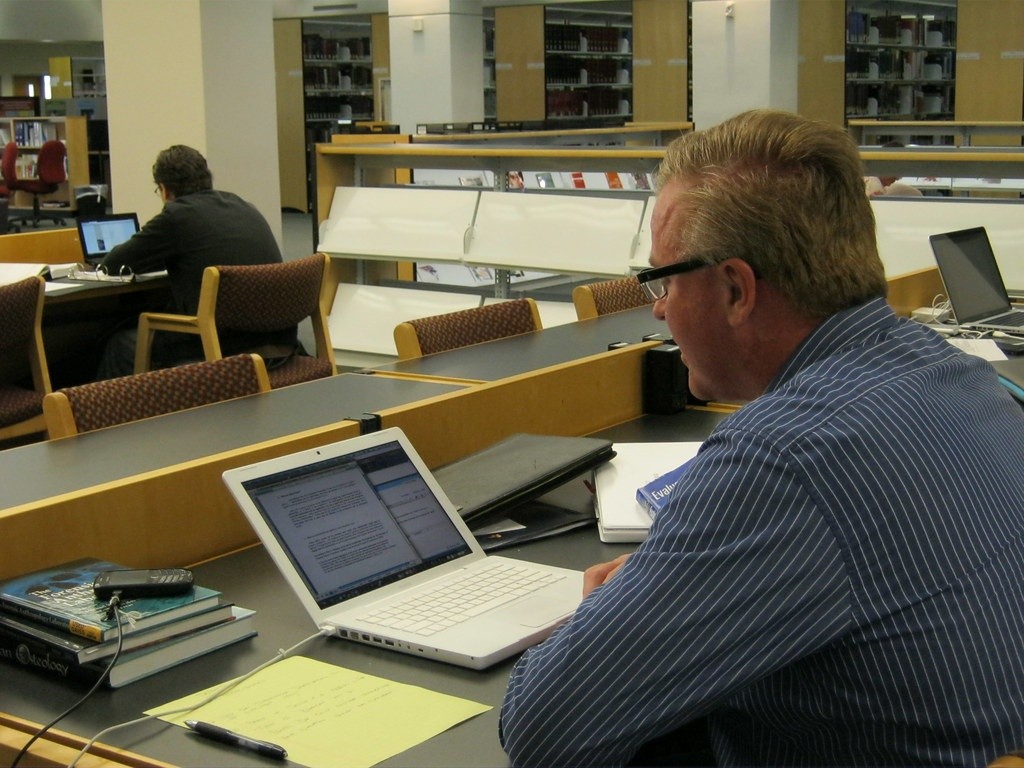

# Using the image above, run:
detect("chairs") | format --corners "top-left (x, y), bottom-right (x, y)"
top-left (0, 254), bottom-right (662, 455)
top-left (12, 138), bottom-right (67, 229)
top-left (0, 141), bottom-right (27, 232)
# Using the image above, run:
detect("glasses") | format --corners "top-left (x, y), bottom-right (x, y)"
top-left (637, 250), bottom-right (763, 302)
top-left (153, 182), bottom-right (172, 198)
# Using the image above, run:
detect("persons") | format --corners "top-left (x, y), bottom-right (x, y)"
top-left (97, 145), bottom-right (299, 380)
top-left (496, 119), bottom-right (1024, 768)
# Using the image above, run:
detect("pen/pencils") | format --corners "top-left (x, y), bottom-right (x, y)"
top-left (183, 720), bottom-right (288, 760)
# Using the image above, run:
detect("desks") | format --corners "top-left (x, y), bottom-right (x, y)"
top-left (43, 267), bottom-right (170, 304)
top-left (1, 374), bottom-right (471, 508)
top-left (1, 409), bottom-right (735, 768)
top-left (362, 300), bottom-right (673, 384)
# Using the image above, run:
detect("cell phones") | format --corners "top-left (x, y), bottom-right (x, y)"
top-left (93, 567), bottom-right (194, 599)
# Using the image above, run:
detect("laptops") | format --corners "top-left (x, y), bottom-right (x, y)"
top-left (221, 427), bottom-right (585, 670)
top-left (76, 212), bottom-right (165, 271)
top-left (928, 227), bottom-right (1024, 335)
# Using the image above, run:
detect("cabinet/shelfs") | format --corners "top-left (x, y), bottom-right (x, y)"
top-left (65, 116), bottom-right (110, 211)
top-left (481, 14), bottom-right (497, 122)
top-left (631, 1), bottom-right (693, 126)
top-left (798, 0), bottom-right (959, 147)
top-left (0, 116), bottom-right (91, 207)
top-left (49, 56), bottom-right (106, 114)
top-left (493, 4), bottom-right (632, 122)
top-left (279, 18), bottom-right (373, 212)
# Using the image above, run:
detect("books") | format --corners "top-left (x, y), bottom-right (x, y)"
top-left (0, 556), bottom-right (259, 687)
top-left (302, 33), bottom-right (374, 200)
top-left (0, 263), bottom-right (78, 286)
top-left (15, 153), bottom-right (68, 180)
top-left (545, 25), bottom-right (619, 118)
top-left (845, 12), bottom-right (957, 145)
top-left (68, 263), bottom-right (168, 282)
top-left (636, 455), bottom-right (695, 522)
top-left (593, 442), bottom-right (705, 544)
top-left (14, 121), bottom-right (57, 147)
